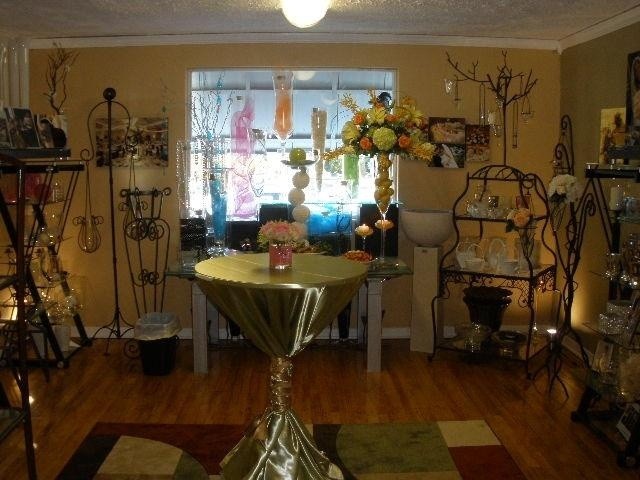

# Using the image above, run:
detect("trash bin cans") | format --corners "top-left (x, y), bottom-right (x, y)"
top-left (134, 312), bottom-right (181, 376)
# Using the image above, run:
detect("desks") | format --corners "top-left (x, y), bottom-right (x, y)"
top-left (166, 251), bottom-right (415, 375)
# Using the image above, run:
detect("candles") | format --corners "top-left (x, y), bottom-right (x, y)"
top-left (359, 223), bottom-right (369, 233)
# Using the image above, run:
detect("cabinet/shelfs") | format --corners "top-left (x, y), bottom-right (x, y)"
top-left (568, 116), bottom-right (637, 468)
top-left (0, 147), bottom-right (93, 480)
top-left (429, 158), bottom-right (554, 381)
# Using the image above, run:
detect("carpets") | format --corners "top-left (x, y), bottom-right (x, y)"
top-left (57, 421), bottom-right (527, 480)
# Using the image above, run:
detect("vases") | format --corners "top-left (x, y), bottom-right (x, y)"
top-left (374, 151), bottom-right (395, 270)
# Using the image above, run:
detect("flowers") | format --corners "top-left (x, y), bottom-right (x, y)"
top-left (319, 86), bottom-right (438, 163)
top-left (256, 219), bottom-right (310, 252)
